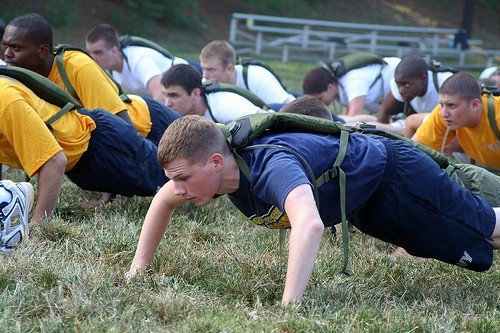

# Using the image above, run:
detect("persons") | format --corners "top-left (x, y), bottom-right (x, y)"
top-left (124, 115), bottom-right (500, 311)
top-left (0, 179), bottom-right (34, 254)
top-left (0, 13), bottom-right (500, 222)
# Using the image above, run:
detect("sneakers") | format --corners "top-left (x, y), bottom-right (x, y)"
top-left (0, 179), bottom-right (34, 255)
